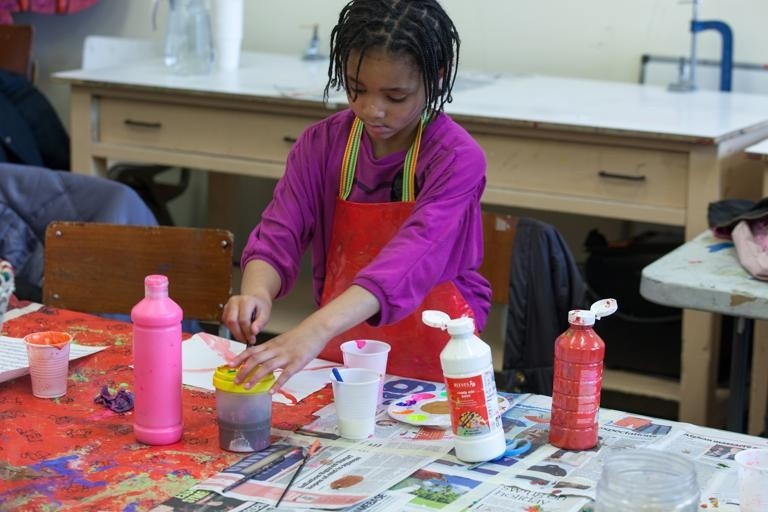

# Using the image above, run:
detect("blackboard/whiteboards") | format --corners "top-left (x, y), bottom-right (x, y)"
top-left (639, 55), bottom-right (768, 94)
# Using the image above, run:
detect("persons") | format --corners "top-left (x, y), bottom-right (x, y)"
top-left (220, 0), bottom-right (492, 395)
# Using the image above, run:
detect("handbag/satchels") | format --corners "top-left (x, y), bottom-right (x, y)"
top-left (580, 229), bottom-right (681, 384)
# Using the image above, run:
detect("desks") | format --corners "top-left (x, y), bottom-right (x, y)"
top-left (0, 294), bottom-right (768, 511)
top-left (635, 224), bottom-right (768, 434)
top-left (45, 61), bottom-right (767, 434)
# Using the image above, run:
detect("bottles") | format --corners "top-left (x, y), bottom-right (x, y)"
top-left (420, 310), bottom-right (507, 464)
top-left (129, 273), bottom-right (184, 445)
top-left (591, 446), bottom-right (702, 512)
top-left (211, 363), bottom-right (273, 454)
top-left (548, 299), bottom-right (621, 455)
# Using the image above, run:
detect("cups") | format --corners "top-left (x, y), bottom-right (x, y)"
top-left (329, 368), bottom-right (379, 441)
top-left (22, 329), bottom-right (69, 399)
top-left (731, 450), bottom-right (768, 512)
top-left (340, 339), bottom-right (391, 408)
top-left (211, 0), bottom-right (246, 85)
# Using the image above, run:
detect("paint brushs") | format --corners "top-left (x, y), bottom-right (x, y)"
top-left (222, 449), bottom-right (301, 493)
top-left (275, 439), bottom-right (321, 508)
top-left (247, 308), bottom-right (256, 349)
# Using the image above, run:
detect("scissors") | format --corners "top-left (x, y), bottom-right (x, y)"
top-left (467, 439), bottom-right (532, 470)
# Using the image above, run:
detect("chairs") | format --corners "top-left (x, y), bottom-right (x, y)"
top-left (476, 207), bottom-right (580, 402)
top-left (41, 218), bottom-right (234, 342)
top-left (0, 21), bottom-right (33, 76)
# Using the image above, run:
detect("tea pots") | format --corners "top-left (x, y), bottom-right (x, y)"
top-left (152, 1), bottom-right (216, 82)
